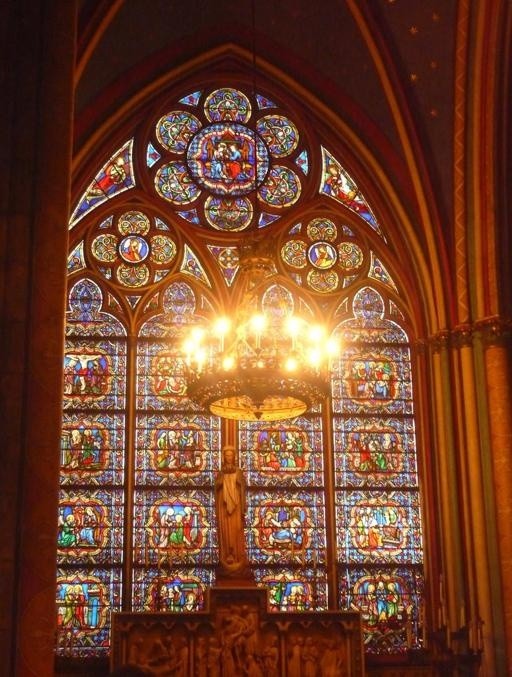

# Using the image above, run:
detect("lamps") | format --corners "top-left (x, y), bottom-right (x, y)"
top-left (182, 3), bottom-right (342, 424)
